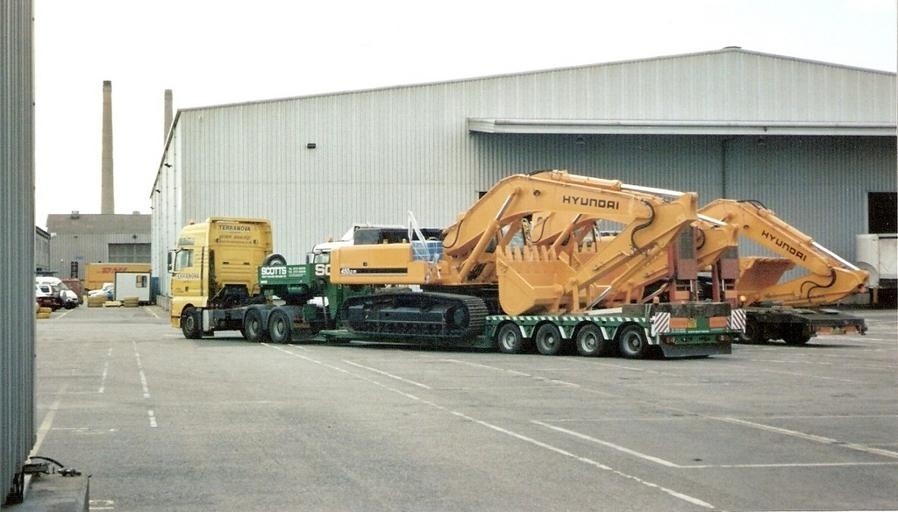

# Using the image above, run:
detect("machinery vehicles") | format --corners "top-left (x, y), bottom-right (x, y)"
top-left (580, 196), bottom-right (871, 315)
top-left (327, 168), bottom-right (742, 319)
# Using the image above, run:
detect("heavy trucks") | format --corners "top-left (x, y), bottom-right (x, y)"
top-left (305, 223), bottom-right (871, 347)
top-left (163, 216), bottom-right (748, 361)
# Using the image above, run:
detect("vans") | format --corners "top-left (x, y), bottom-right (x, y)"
top-left (35, 276), bottom-right (79, 310)
